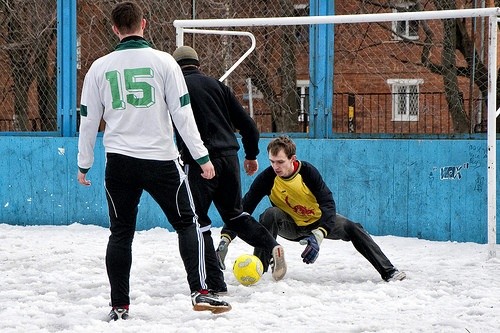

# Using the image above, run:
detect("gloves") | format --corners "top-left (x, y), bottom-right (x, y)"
top-left (215, 238), bottom-right (231, 270)
top-left (299, 228), bottom-right (324, 264)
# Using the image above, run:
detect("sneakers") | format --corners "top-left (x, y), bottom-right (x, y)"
top-left (208, 287), bottom-right (227, 295)
top-left (385, 270), bottom-right (405, 281)
top-left (269, 244), bottom-right (287, 281)
top-left (108, 307), bottom-right (128, 321)
top-left (190, 291), bottom-right (232, 314)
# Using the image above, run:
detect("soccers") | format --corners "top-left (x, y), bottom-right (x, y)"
top-left (233, 255), bottom-right (263, 286)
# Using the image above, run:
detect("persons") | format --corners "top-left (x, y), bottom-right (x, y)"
top-left (172, 46), bottom-right (286, 295)
top-left (216, 137), bottom-right (405, 282)
top-left (77, 2), bottom-right (232, 320)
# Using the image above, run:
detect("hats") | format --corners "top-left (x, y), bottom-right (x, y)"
top-left (173, 46), bottom-right (200, 70)
